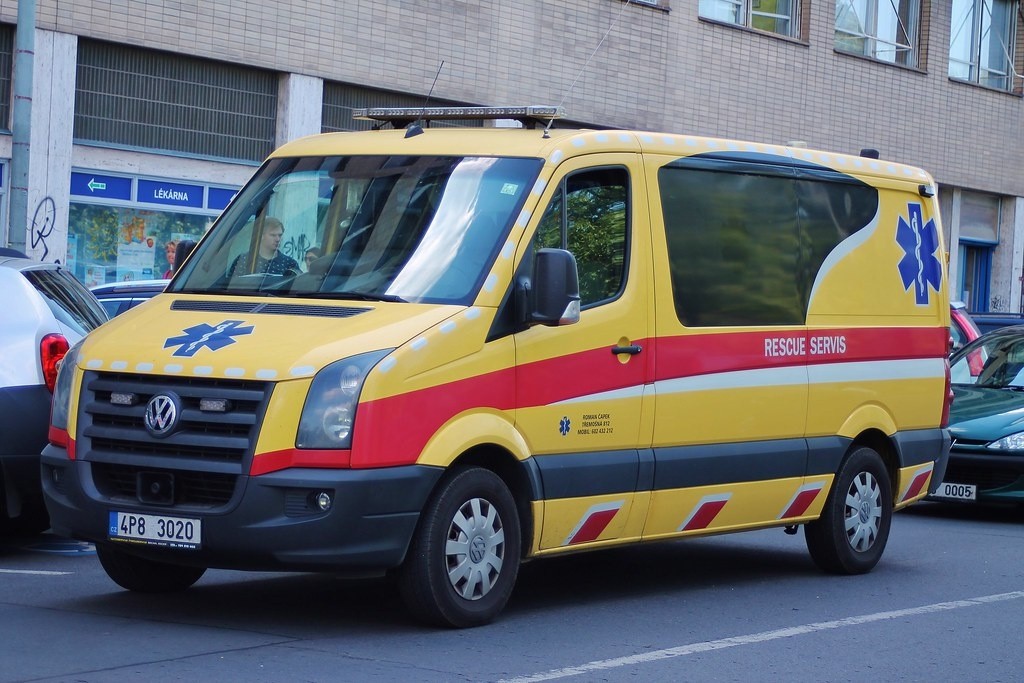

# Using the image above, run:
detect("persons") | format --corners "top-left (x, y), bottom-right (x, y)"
top-left (228, 217), bottom-right (303, 276)
top-left (163, 239), bottom-right (178, 279)
top-left (305, 247), bottom-right (322, 271)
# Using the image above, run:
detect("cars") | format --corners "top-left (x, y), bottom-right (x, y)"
top-left (922, 324), bottom-right (1024, 517)
top-left (946, 301), bottom-right (988, 385)
top-left (89, 278), bottom-right (172, 323)
top-left (0, 250), bottom-right (110, 547)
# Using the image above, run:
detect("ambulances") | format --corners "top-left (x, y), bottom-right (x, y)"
top-left (39, 104), bottom-right (955, 630)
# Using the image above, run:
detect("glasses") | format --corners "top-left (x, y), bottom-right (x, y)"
top-left (305, 256), bottom-right (318, 262)
